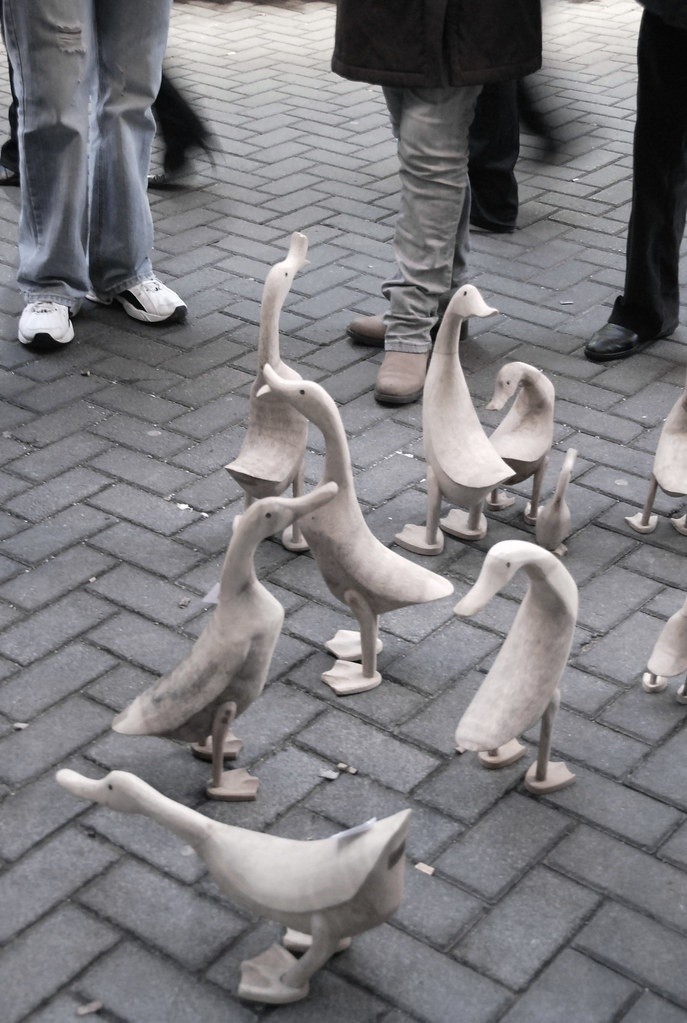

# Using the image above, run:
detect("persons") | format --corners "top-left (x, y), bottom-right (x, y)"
top-left (0, 1), bottom-right (224, 350)
top-left (320, 0), bottom-right (687, 403)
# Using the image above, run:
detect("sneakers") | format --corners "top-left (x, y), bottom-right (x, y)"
top-left (19, 300), bottom-right (75, 350)
top-left (85, 274), bottom-right (188, 326)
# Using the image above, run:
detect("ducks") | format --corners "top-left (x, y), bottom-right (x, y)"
top-left (52, 227), bottom-right (687, 1004)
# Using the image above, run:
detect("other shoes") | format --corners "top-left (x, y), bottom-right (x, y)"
top-left (0, 164), bottom-right (20, 186)
top-left (346, 314), bottom-right (469, 341)
top-left (148, 126), bottom-right (214, 190)
top-left (374, 350), bottom-right (429, 403)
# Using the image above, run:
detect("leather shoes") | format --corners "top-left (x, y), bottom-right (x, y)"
top-left (584, 323), bottom-right (666, 360)
